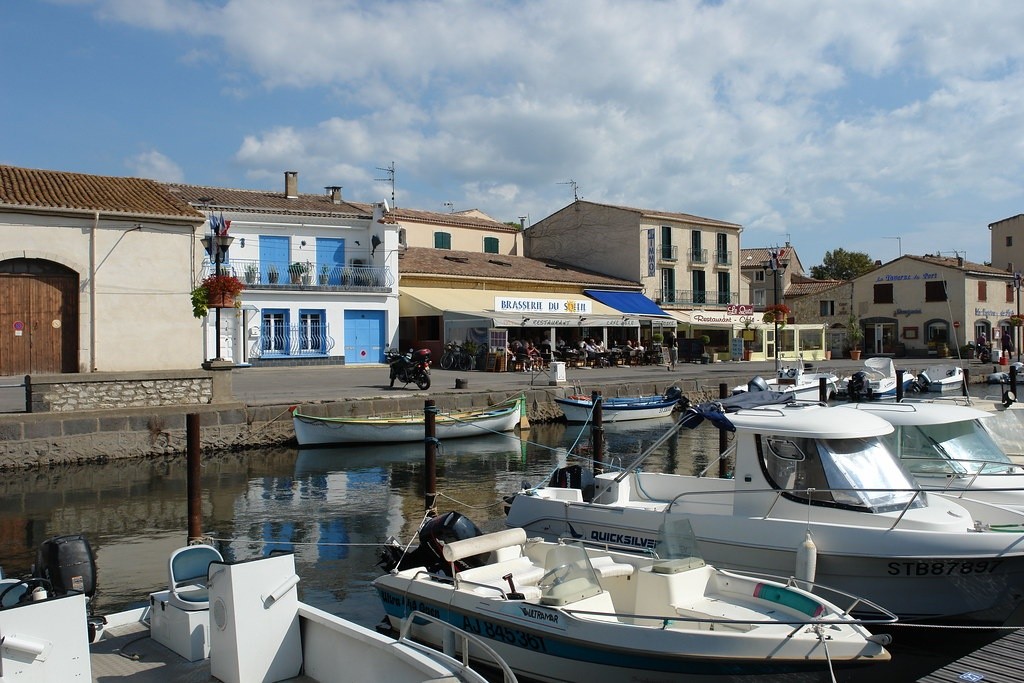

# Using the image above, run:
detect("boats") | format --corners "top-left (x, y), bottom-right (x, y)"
top-left (732, 340), bottom-right (840, 405)
top-left (0, 530), bottom-right (519, 683)
top-left (988, 362), bottom-right (1024, 384)
top-left (838, 356), bottom-right (915, 399)
top-left (555, 386), bottom-right (691, 424)
top-left (291, 394), bottom-right (530, 450)
top-left (505, 388), bottom-right (1024, 652)
top-left (913, 363), bottom-right (965, 392)
top-left (372, 502), bottom-right (899, 683)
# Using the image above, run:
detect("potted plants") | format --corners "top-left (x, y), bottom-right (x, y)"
top-left (319, 264), bottom-right (329, 284)
top-left (244, 265), bottom-right (258, 284)
top-left (844, 314), bottom-right (865, 360)
top-left (266, 264), bottom-right (280, 284)
top-left (221, 264), bottom-right (232, 276)
top-left (354, 272), bottom-right (372, 286)
top-left (959, 336), bottom-right (975, 359)
top-left (459, 339), bottom-right (479, 370)
top-left (826, 339), bottom-right (832, 360)
top-left (286, 262), bottom-right (308, 285)
top-left (744, 346), bottom-right (754, 361)
top-left (301, 261), bottom-right (314, 285)
top-left (651, 333), bottom-right (664, 364)
top-left (341, 267), bottom-right (352, 285)
top-left (698, 334), bottom-right (710, 364)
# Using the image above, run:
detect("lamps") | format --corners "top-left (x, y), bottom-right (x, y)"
top-left (623, 316), bottom-right (630, 322)
top-left (522, 315), bottom-right (530, 322)
top-left (579, 315), bottom-right (586, 322)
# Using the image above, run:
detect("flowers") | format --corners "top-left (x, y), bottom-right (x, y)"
top-left (189, 275), bottom-right (243, 325)
top-left (761, 303), bottom-right (791, 332)
top-left (1010, 314), bottom-right (1024, 327)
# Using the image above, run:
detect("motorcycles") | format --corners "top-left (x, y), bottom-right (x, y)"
top-left (977, 340), bottom-right (990, 362)
top-left (383, 345), bottom-right (432, 390)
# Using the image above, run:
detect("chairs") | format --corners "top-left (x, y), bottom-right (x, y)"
top-left (168, 543), bottom-right (225, 612)
top-left (511, 347), bottom-right (660, 372)
top-left (0, 579), bottom-right (29, 608)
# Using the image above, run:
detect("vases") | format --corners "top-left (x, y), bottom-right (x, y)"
top-left (207, 291), bottom-right (236, 308)
top-left (1011, 319), bottom-right (1024, 326)
top-left (772, 310), bottom-right (783, 322)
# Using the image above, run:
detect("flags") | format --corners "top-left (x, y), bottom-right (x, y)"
top-left (209, 211), bottom-right (225, 232)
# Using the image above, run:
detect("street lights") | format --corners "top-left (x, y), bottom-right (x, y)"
top-left (1005, 273), bottom-right (1024, 362)
top-left (200, 236), bottom-right (235, 360)
top-left (761, 259), bottom-right (790, 372)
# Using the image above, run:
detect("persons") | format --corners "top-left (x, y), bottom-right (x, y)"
top-left (1001, 331), bottom-right (1013, 359)
top-left (506, 335), bottom-right (646, 373)
top-left (977, 332), bottom-right (986, 346)
top-left (668, 332), bottom-right (678, 371)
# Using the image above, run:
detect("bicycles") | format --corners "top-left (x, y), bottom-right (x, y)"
top-left (440, 340), bottom-right (476, 371)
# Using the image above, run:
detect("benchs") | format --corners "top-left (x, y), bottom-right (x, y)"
top-left (675, 591), bottom-right (806, 635)
top-left (443, 527), bottom-right (546, 598)
